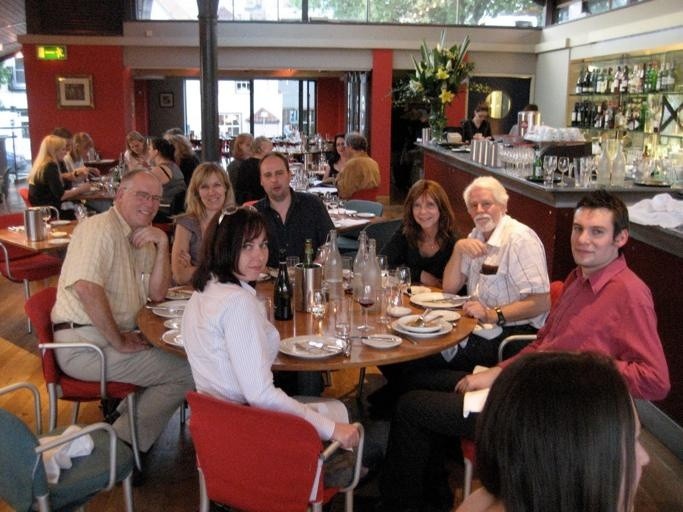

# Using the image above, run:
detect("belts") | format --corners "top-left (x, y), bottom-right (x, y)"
top-left (54, 322), bottom-right (82, 330)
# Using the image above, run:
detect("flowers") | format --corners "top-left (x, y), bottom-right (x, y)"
top-left (380, 25), bottom-right (492, 144)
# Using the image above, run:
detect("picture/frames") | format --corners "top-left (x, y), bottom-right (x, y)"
top-left (158, 91), bottom-right (174, 108)
top-left (55, 72), bottom-right (95, 111)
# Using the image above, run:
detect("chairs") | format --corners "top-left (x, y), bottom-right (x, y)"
top-left (185, 388), bottom-right (365, 512)
top-left (3, 156), bottom-right (414, 272)
top-left (462, 336), bottom-right (539, 506)
top-left (2, 382), bottom-right (134, 512)
top-left (22, 286), bottom-right (190, 481)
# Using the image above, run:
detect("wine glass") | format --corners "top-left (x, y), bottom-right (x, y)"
top-left (39, 206), bottom-right (51, 234)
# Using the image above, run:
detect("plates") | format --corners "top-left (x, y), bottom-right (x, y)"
top-left (50, 219), bottom-right (70, 226)
top-left (151, 284), bottom-right (466, 358)
top-left (50, 231), bottom-right (66, 237)
top-left (46, 239), bottom-right (69, 244)
top-left (356, 212), bottom-right (375, 218)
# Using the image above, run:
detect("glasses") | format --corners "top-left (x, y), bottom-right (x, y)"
top-left (218, 205), bottom-right (257, 225)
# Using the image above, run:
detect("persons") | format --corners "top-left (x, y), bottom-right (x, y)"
top-left (28, 126), bottom-right (670, 510)
top-left (412, 110), bottom-right (430, 140)
top-left (509, 104), bottom-right (538, 135)
top-left (463, 105), bottom-right (494, 144)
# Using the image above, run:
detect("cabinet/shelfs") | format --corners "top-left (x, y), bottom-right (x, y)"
top-left (564, 45), bottom-right (682, 139)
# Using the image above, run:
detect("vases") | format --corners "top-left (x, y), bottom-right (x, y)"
top-left (429, 103), bottom-right (444, 147)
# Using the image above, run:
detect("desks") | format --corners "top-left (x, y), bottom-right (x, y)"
top-left (419, 156), bottom-right (565, 283)
top-left (137, 269), bottom-right (477, 374)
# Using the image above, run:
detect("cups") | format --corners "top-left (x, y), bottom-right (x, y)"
top-left (499, 135), bottom-right (672, 188)
top-left (421, 128), bottom-right (431, 145)
top-left (24, 209), bottom-right (45, 242)
top-left (289, 152), bottom-right (355, 219)
top-left (75, 175), bottom-right (120, 198)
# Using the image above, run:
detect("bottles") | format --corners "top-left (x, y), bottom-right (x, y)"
top-left (570, 95), bottom-right (645, 131)
top-left (273, 228), bottom-right (411, 339)
top-left (575, 59), bottom-right (675, 93)
